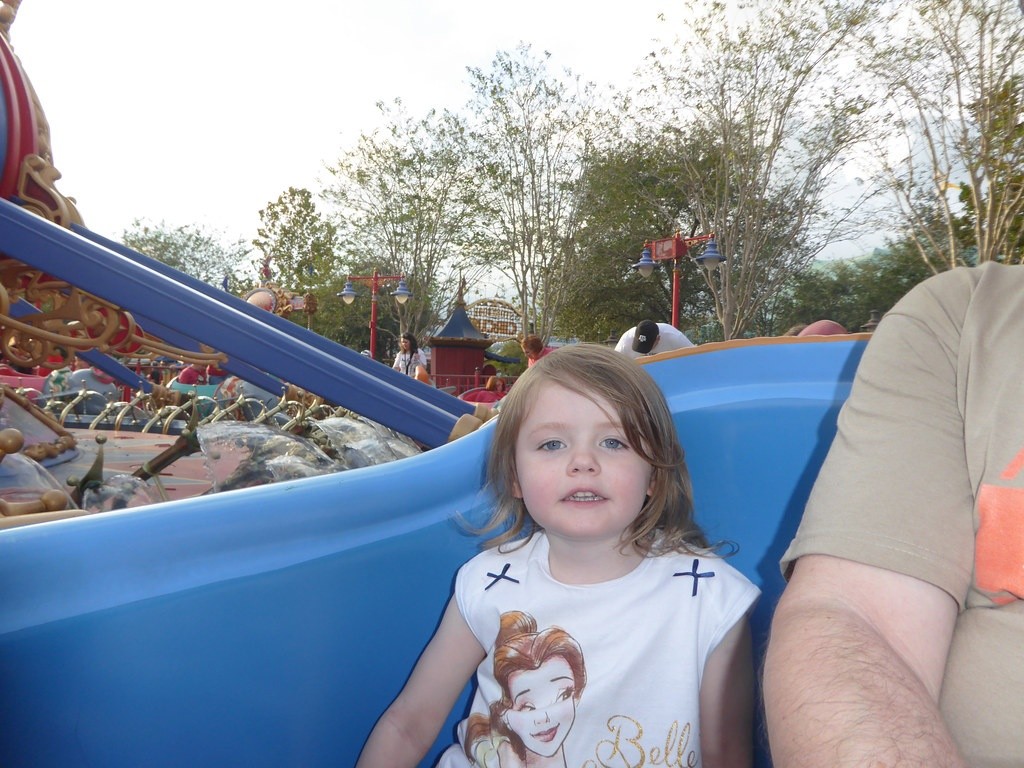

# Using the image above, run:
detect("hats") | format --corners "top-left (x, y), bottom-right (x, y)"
top-left (632, 320), bottom-right (659, 354)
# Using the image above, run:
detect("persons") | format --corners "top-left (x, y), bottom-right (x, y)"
top-left (763, 259), bottom-right (1024, 768)
top-left (360, 332), bottom-right (560, 401)
top-left (614, 319), bottom-right (699, 359)
top-left (356, 343), bottom-right (764, 768)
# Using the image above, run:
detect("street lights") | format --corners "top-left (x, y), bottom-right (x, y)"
top-left (335, 268), bottom-right (420, 365)
top-left (630, 230), bottom-right (728, 331)
